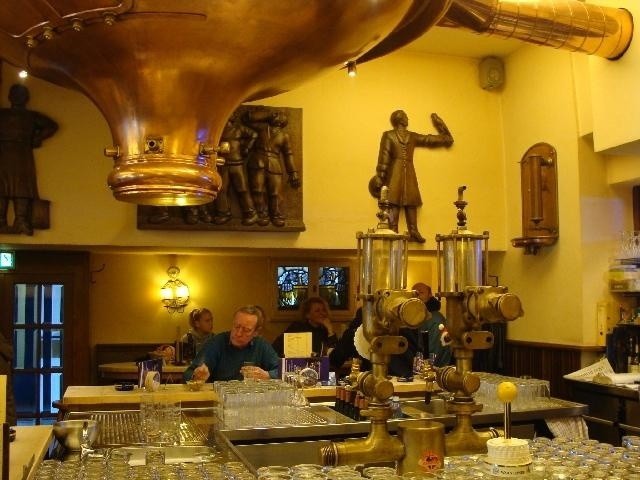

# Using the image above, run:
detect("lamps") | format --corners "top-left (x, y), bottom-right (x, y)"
top-left (0, 252), bottom-right (14, 269)
top-left (347, 58), bottom-right (358, 78)
top-left (158, 263), bottom-right (192, 313)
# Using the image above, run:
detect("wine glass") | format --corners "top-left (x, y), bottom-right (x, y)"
top-left (614, 229), bottom-right (640, 258)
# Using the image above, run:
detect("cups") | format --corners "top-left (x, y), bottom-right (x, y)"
top-left (213, 361), bottom-right (299, 427)
top-left (140, 393), bottom-right (182, 443)
top-left (33, 436), bottom-right (640, 480)
top-left (472, 372), bottom-right (551, 412)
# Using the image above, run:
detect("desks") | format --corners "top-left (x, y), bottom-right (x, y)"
top-left (0, 361), bottom-right (440, 480)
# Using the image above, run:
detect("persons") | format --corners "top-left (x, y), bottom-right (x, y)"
top-left (172, 306), bottom-right (218, 363)
top-left (329, 302), bottom-right (416, 378)
top-left (147, 205), bottom-right (200, 224)
top-left (0, 83), bottom-right (60, 233)
top-left (211, 110), bottom-right (263, 225)
top-left (183, 305), bottom-right (283, 380)
top-left (241, 104), bottom-right (301, 228)
top-left (272, 296), bottom-right (338, 359)
top-left (406, 283), bottom-right (454, 367)
top-left (377, 109), bottom-right (454, 242)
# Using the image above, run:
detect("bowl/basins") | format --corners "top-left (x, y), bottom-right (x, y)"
top-left (185, 379), bottom-right (205, 392)
top-left (53, 419), bottom-right (101, 452)
